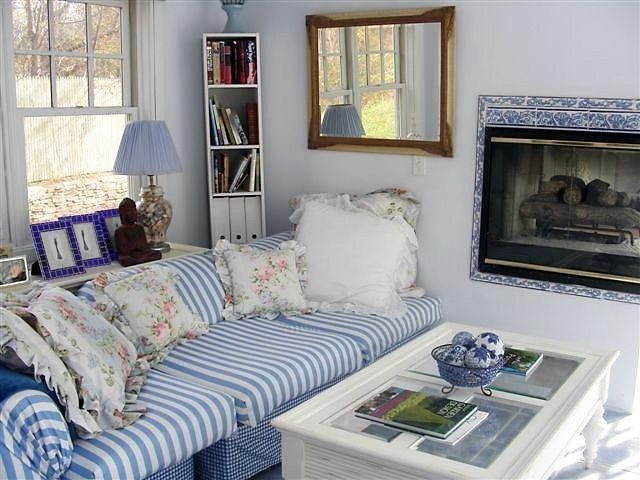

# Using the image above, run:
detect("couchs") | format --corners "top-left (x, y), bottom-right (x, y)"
top-left (0, 228), bottom-right (444, 480)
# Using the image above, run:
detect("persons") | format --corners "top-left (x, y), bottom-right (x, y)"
top-left (115, 197), bottom-right (163, 267)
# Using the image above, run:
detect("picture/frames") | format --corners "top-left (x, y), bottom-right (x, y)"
top-left (95, 209), bottom-right (122, 261)
top-left (29, 220), bottom-right (86, 280)
top-left (58, 213), bottom-right (111, 269)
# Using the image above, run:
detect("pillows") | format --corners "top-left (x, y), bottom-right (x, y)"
top-left (9, 282), bottom-right (150, 429)
top-left (94, 271), bottom-right (209, 367)
top-left (0, 299), bottom-right (102, 441)
top-left (213, 239), bottom-right (315, 321)
top-left (288, 186), bottom-right (421, 318)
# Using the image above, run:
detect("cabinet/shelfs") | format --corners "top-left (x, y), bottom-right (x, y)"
top-left (202, 32), bottom-right (267, 249)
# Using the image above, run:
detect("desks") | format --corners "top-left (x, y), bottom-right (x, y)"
top-left (271, 320), bottom-right (619, 480)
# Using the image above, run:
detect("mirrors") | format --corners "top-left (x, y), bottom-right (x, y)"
top-left (305, 5), bottom-right (455, 159)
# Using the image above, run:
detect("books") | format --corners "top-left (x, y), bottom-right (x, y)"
top-left (353, 385), bottom-right (491, 446)
top-left (499, 346), bottom-right (543, 378)
top-left (206, 39), bottom-right (260, 197)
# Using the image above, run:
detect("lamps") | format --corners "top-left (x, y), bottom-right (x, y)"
top-left (113, 120), bottom-right (180, 252)
top-left (319, 104), bottom-right (366, 138)
